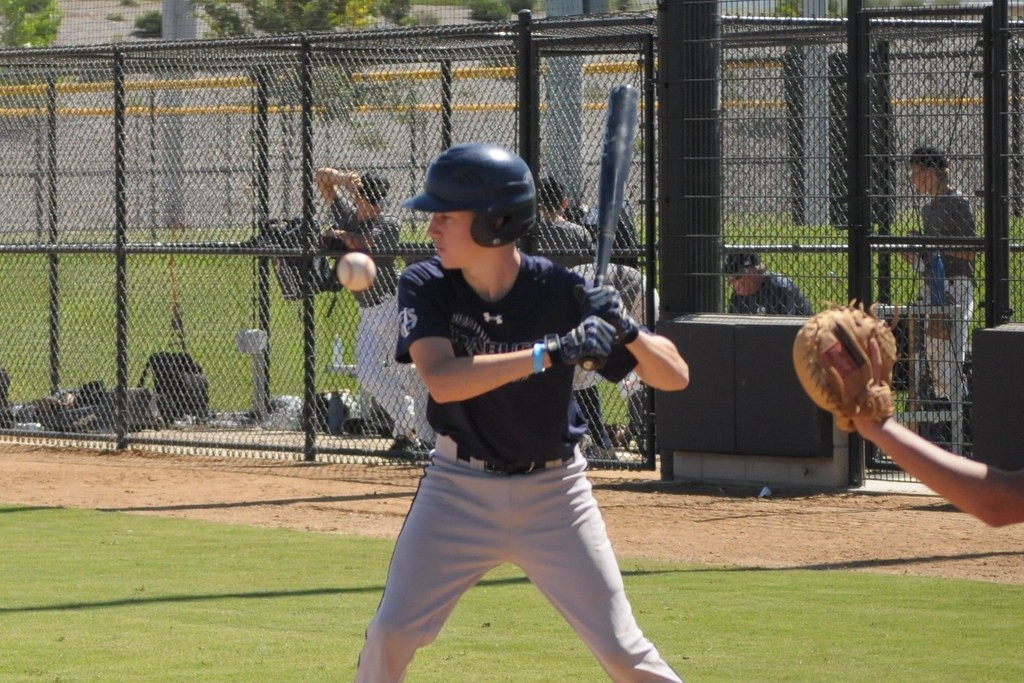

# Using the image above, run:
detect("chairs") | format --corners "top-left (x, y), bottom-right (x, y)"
top-left (873, 304), bottom-right (963, 456)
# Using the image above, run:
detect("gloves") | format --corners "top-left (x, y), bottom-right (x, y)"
top-left (533, 315), bottom-right (616, 374)
top-left (573, 284), bottom-right (638, 344)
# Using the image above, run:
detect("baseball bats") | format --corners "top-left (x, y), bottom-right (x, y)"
top-left (575, 84), bottom-right (640, 370)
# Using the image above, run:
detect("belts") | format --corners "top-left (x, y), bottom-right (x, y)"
top-left (456, 443), bottom-right (574, 477)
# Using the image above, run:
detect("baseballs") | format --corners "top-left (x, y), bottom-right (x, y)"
top-left (336, 252), bottom-right (377, 292)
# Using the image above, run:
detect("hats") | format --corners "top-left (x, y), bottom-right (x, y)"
top-left (359, 172), bottom-right (391, 208)
top-left (719, 247), bottom-right (762, 278)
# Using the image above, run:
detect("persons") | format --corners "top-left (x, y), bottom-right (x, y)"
top-left (314, 168), bottom-right (436, 452)
top-left (536, 175), bottom-right (658, 457)
top-left (352, 143), bottom-right (690, 683)
top-left (832, 336), bottom-right (1024, 527)
top-left (901, 146), bottom-right (975, 400)
top-left (722, 252), bottom-right (811, 316)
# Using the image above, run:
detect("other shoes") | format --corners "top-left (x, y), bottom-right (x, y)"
top-left (385, 437), bottom-right (412, 456)
top-left (413, 440), bottom-right (438, 454)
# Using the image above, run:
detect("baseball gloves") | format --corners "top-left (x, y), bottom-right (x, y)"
top-left (790, 305), bottom-right (898, 431)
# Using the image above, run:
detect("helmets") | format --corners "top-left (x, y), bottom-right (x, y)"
top-left (399, 143), bottom-right (537, 248)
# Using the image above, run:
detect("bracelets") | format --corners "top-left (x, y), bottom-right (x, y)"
top-left (531, 342), bottom-right (544, 375)
